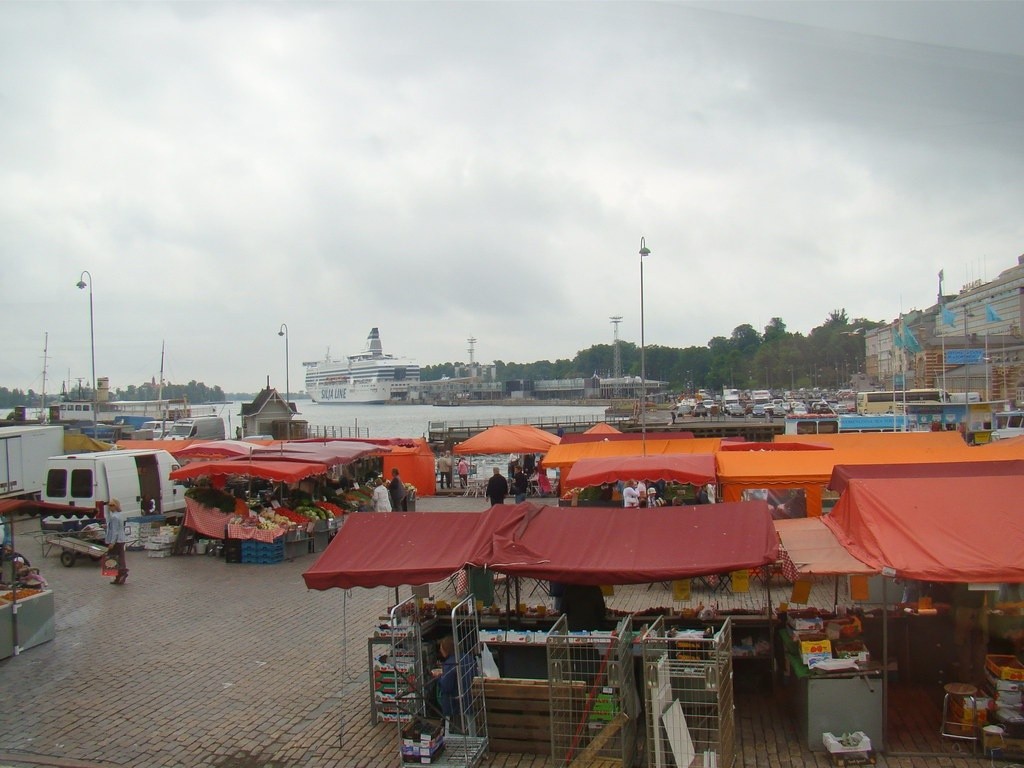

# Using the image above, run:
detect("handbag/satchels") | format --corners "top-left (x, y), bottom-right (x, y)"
top-left (102, 550), bottom-right (119, 576)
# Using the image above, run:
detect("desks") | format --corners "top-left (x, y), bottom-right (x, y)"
top-left (478, 641), bottom-right (595, 646)
top-left (474, 479), bottom-right (490, 497)
top-left (436, 616), bottom-right (567, 672)
top-left (866, 611), bottom-right (956, 683)
top-left (778, 628), bottom-right (883, 751)
top-left (610, 613), bottom-right (781, 704)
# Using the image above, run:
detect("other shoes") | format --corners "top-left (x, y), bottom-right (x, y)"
top-left (110, 573), bottom-right (128, 584)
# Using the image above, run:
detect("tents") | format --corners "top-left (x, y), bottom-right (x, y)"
top-left (165, 423), bottom-right (1024, 758)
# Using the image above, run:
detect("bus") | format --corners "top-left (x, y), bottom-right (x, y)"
top-left (856, 389), bottom-right (952, 416)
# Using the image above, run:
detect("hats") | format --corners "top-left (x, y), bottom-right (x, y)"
top-left (647, 488), bottom-right (656, 495)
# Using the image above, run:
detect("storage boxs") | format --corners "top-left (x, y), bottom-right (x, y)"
top-left (479, 629), bottom-right (725, 676)
top-left (786, 608), bottom-right (871, 665)
top-left (225, 505), bottom-right (369, 564)
top-left (731, 637), bottom-right (773, 661)
top-left (886, 656), bottom-right (899, 682)
top-left (822, 731), bottom-right (877, 765)
top-left (373, 612), bottom-right (437, 724)
top-left (587, 692), bottom-right (620, 720)
top-left (949, 654), bottom-right (1024, 760)
top-left (401, 715), bottom-right (445, 764)
top-left (79, 515), bottom-right (181, 558)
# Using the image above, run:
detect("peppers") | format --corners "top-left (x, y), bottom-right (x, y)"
top-left (274, 507), bottom-right (307, 523)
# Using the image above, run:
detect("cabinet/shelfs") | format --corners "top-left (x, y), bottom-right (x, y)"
top-left (368, 621), bottom-right (439, 725)
top-left (642, 614), bottom-right (738, 768)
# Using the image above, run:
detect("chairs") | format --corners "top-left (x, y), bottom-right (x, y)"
top-left (460, 478), bottom-right (476, 496)
top-left (529, 478), bottom-right (542, 497)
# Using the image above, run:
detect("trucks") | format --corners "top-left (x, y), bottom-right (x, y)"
top-left (0, 424), bottom-right (64, 500)
top-left (723, 389), bottom-right (740, 395)
top-left (751, 390), bottom-right (770, 405)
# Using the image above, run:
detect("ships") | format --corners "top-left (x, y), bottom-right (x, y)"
top-left (305, 327), bottom-right (420, 404)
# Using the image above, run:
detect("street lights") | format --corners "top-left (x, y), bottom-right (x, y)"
top-left (639, 237), bottom-right (650, 457)
top-left (963, 303), bottom-right (976, 442)
top-left (77, 270), bottom-right (97, 441)
top-left (279, 323), bottom-right (290, 441)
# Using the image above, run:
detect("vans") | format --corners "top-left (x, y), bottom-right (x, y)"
top-left (991, 412), bottom-right (1024, 439)
top-left (138, 420), bottom-right (175, 431)
top-left (41, 448), bottom-right (195, 533)
top-left (724, 395), bottom-right (740, 412)
top-left (163, 415), bottom-right (225, 441)
top-left (243, 435), bottom-right (275, 441)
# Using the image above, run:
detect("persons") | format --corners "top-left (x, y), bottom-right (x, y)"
top-left (511, 465), bottom-right (529, 503)
top-left (537, 455), bottom-right (552, 493)
top-left (430, 635), bottom-right (477, 734)
top-left (699, 483), bottom-right (711, 504)
top-left (437, 449), bottom-right (451, 488)
top-left (485, 467), bottom-right (508, 508)
top-left (443, 450), bottom-right (454, 488)
top-left (390, 467), bottom-right (404, 511)
top-left (707, 482), bottom-right (716, 504)
top-left (646, 487), bottom-right (657, 508)
top-left (370, 478), bottom-right (392, 512)
top-left (105, 499), bottom-right (129, 584)
top-left (509, 452), bottom-right (520, 465)
top-left (458, 456), bottom-right (468, 488)
top-left (623, 479), bottom-right (640, 508)
top-left (638, 479), bottom-right (647, 506)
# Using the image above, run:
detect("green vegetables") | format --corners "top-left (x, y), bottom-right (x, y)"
top-left (184, 486), bottom-right (237, 515)
top-left (288, 488), bottom-right (329, 521)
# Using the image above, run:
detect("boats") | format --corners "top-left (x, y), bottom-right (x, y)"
top-left (325, 377), bottom-right (347, 383)
top-left (56, 378), bottom-right (192, 423)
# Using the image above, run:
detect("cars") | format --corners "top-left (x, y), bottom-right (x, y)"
top-left (154, 431), bottom-right (169, 440)
top-left (677, 388), bottom-right (858, 416)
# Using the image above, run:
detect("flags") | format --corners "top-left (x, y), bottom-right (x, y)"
top-left (894, 329), bottom-right (902, 348)
top-left (985, 307), bottom-right (1000, 323)
top-left (941, 307), bottom-right (958, 327)
top-left (903, 323), bottom-right (922, 353)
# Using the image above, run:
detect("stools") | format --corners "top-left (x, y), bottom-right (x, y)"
top-left (444, 698), bottom-right (482, 735)
top-left (981, 724), bottom-right (1007, 767)
top-left (940, 683), bottom-right (978, 754)
